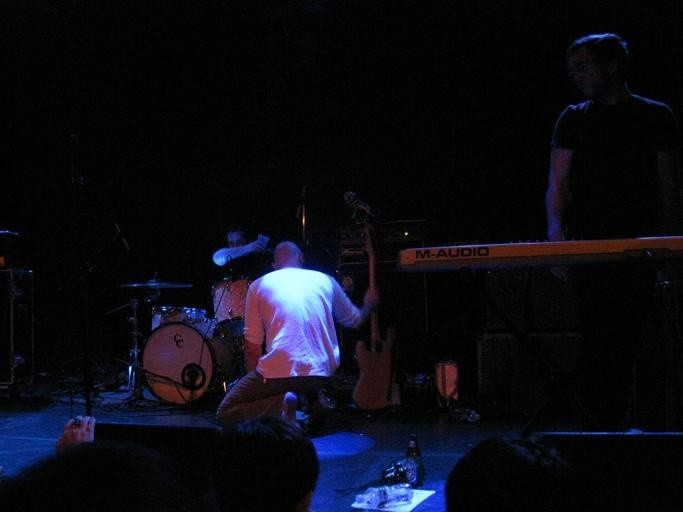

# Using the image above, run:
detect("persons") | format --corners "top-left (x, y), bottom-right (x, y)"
top-left (444, 430), bottom-right (581, 510)
top-left (211, 238), bottom-right (378, 436)
top-left (210, 417), bottom-right (319, 512)
top-left (544, 32), bottom-right (682, 426)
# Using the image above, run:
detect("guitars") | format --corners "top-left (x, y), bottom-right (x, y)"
top-left (352, 222), bottom-right (404, 410)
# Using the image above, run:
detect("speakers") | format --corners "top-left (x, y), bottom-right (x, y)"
top-left (0, 269), bottom-right (35, 385)
top-left (337, 258), bottom-right (432, 390)
top-left (96, 424), bottom-right (219, 467)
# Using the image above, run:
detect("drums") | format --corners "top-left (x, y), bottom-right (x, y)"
top-left (150, 304), bottom-right (179, 330)
top-left (217, 316), bottom-right (244, 354)
top-left (139, 319), bottom-right (232, 408)
top-left (177, 305), bottom-right (206, 333)
top-left (211, 276), bottom-right (259, 325)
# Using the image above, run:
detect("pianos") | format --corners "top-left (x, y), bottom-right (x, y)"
top-left (400, 232), bottom-right (683, 274)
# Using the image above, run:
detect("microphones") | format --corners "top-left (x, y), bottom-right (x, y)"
top-left (344, 191), bottom-right (372, 213)
top-left (109, 214), bottom-right (129, 251)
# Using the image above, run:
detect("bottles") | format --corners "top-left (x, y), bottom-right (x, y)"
top-left (354, 482), bottom-right (414, 509)
top-left (406, 432), bottom-right (425, 487)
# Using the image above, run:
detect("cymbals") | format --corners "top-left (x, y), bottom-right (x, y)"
top-left (117, 279), bottom-right (192, 290)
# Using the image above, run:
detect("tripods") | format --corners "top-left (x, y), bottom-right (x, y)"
top-left (105, 293), bottom-right (163, 411)
